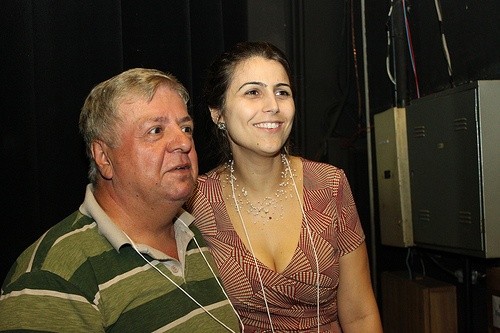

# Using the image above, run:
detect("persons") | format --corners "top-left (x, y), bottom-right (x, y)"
top-left (2, 69), bottom-right (243, 333)
top-left (184, 40), bottom-right (383, 332)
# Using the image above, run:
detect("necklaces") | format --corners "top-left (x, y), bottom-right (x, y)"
top-left (119, 216), bottom-right (243, 332)
top-left (223, 154), bottom-right (321, 333)
